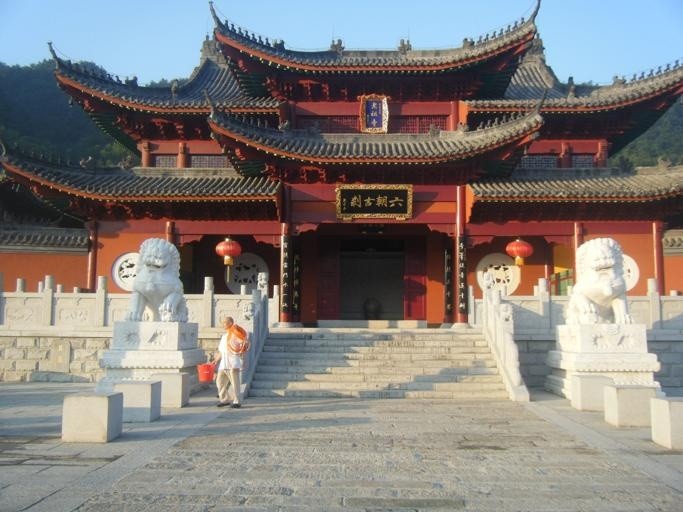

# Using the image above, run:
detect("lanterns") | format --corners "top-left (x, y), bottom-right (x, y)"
top-left (215, 237), bottom-right (242, 283)
top-left (505, 237), bottom-right (534, 266)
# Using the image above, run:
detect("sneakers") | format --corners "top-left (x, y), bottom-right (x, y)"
top-left (216, 403), bottom-right (241, 408)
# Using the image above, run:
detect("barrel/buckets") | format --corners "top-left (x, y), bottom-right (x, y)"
top-left (227, 325), bottom-right (249, 356)
top-left (196, 362), bottom-right (217, 382)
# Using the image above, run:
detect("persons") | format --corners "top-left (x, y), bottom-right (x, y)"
top-left (211, 316), bottom-right (241, 408)
